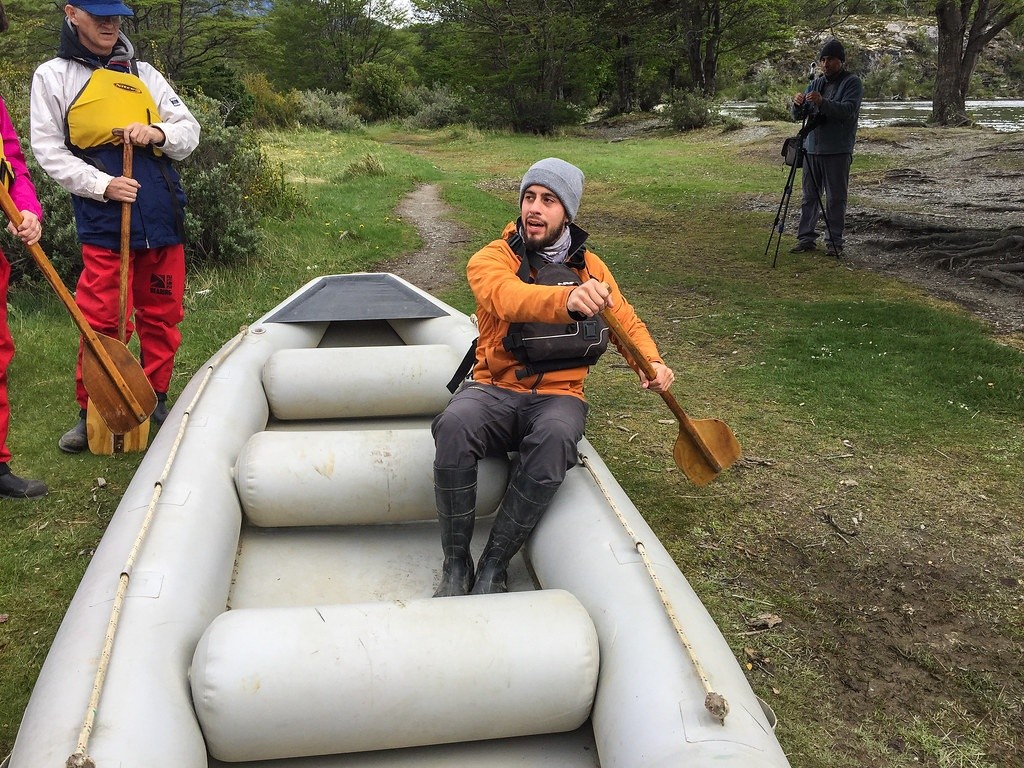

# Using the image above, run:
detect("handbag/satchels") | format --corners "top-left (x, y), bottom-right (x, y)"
top-left (780, 136), bottom-right (804, 174)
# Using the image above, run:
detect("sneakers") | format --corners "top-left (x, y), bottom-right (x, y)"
top-left (151, 393), bottom-right (169, 425)
top-left (790, 243), bottom-right (816, 254)
top-left (826, 246), bottom-right (842, 256)
top-left (0, 471), bottom-right (46, 500)
top-left (59, 418), bottom-right (88, 453)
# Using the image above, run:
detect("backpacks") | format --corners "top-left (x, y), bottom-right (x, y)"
top-left (502, 234), bottom-right (608, 380)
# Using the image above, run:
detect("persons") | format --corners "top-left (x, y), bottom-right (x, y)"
top-left (790, 38), bottom-right (864, 256)
top-left (430, 157), bottom-right (676, 598)
top-left (30, 0), bottom-right (201, 454)
top-left (0, 0), bottom-right (43, 502)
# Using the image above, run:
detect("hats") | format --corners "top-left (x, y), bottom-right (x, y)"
top-left (68, 0), bottom-right (134, 16)
top-left (820, 38), bottom-right (845, 63)
top-left (519, 157), bottom-right (585, 226)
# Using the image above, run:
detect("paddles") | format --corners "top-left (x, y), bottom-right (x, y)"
top-left (600, 307), bottom-right (740, 488)
top-left (87, 129), bottom-right (149, 455)
top-left (0, 182), bottom-right (157, 434)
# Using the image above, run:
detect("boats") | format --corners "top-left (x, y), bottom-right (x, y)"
top-left (7, 271), bottom-right (792, 768)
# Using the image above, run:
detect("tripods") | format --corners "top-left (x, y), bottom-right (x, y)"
top-left (764, 102), bottom-right (840, 269)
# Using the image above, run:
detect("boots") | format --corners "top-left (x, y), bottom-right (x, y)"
top-left (474, 463), bottom-right (557, 595)
top-left (431, 459), bottom-right (479, 597)
